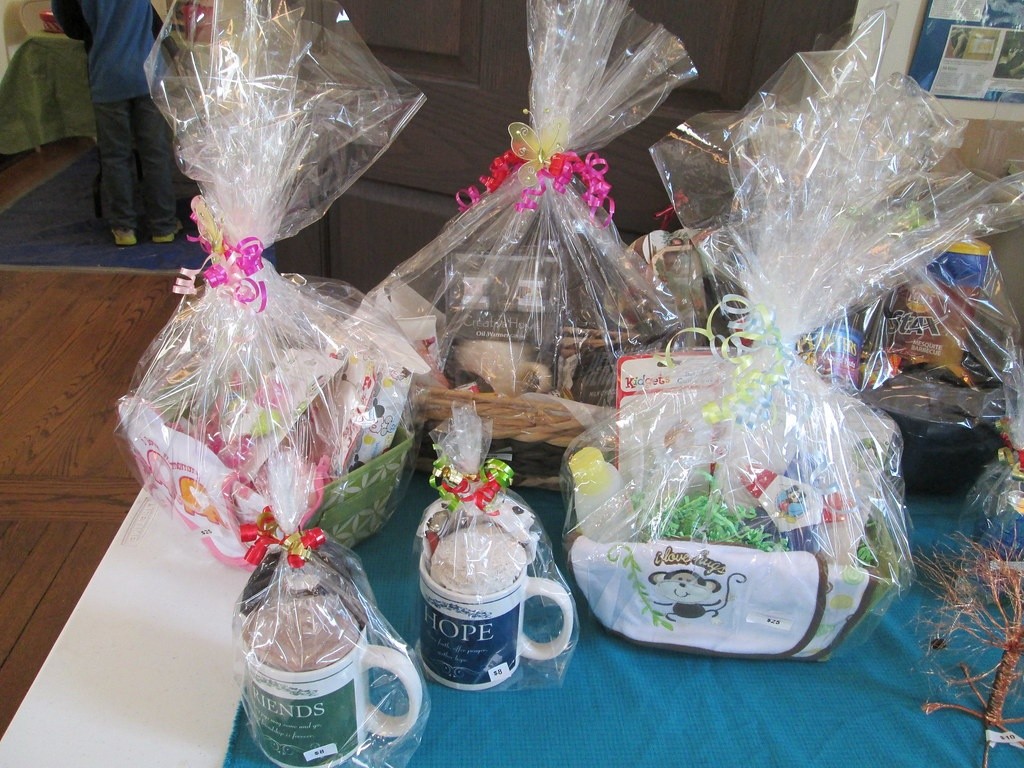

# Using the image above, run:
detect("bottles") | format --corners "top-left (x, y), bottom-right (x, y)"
top-left (568, 447), bottom-right (636, 543)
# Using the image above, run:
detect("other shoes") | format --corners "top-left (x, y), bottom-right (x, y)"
top-left (111, 224), bottom-right (137, 245)
top-left (152, 218), bottom-right (181, 243)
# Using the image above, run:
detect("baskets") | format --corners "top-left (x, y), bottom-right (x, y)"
top-left (406, 323), bottom-right (678, 489)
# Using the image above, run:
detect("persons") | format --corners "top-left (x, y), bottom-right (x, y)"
top-left (52, 0), bottom-right (184, 245)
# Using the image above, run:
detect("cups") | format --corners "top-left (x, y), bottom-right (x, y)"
top-left (247, 626), bottom-right (423, 768)
top-left (419, 552), bottom-right (573, 691)
top-left (455, 339), bottom-right (554, 394)
